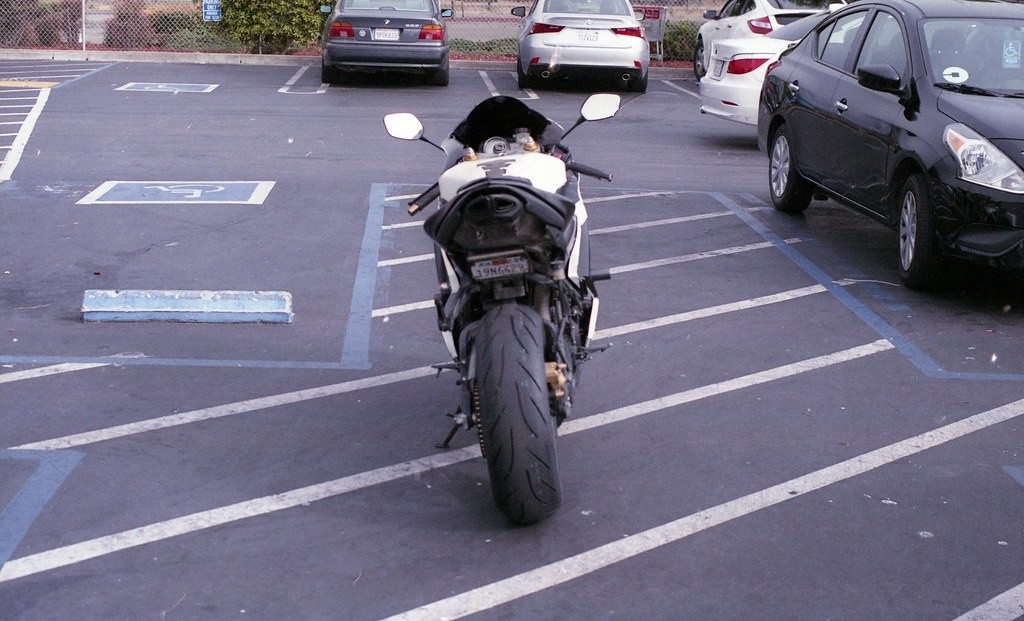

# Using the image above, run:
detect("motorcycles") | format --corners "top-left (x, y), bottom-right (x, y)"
top-left (383, 81), bottom-right (620, 524)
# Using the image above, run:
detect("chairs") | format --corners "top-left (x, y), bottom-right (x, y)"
top-left (599, 1), bottom-right (616, 14)
top-left (828, 25), bottom-right (889, 71)
top-left (404, 0), bottom-right (419, 9)
top-left (351, 0), bottom-right (371, 7)
top-left (553, 0), bottom-right (570, 13)
top-left (888, 33), bottom-right (907, 74)
top-left (930, 27), bottom-right (981, 81)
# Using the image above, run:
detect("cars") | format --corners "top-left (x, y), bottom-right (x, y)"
top-left (700, 4), bottom-right (902, 126)
top-left (509, 0), bottom-right (651, 94)
top-left (693, 0), bottom-right (850, 84)
top-left (754, 0), bottom-right (1024, 295)
top-left (319, 2), bottom-right (454, 88)
